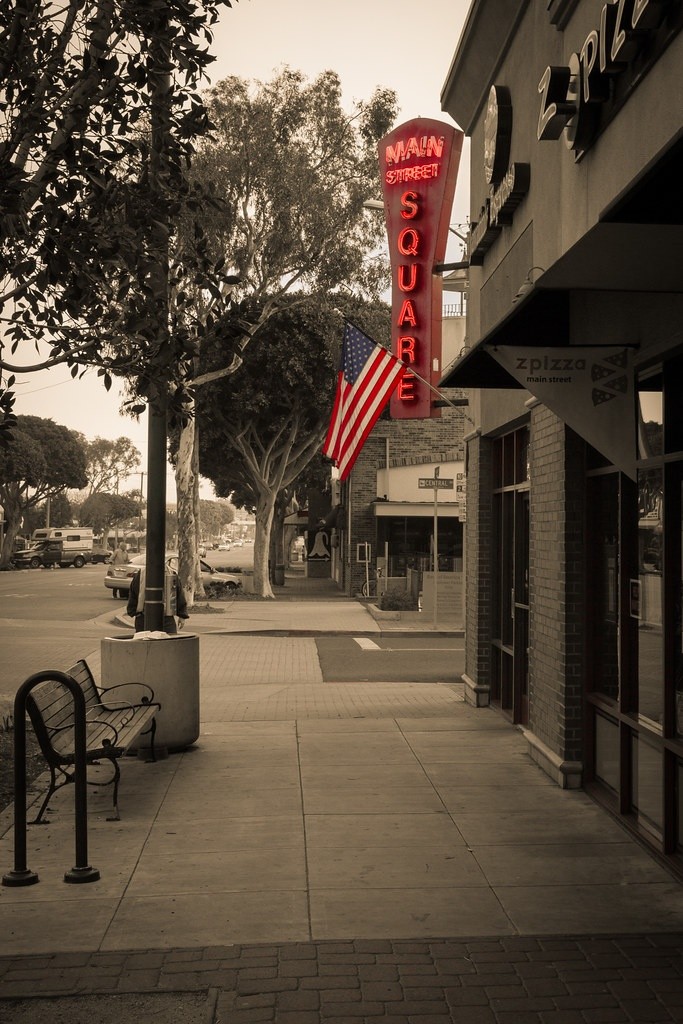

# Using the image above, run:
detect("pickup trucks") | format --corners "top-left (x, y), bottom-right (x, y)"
top-left (11, 540), bottom-right (92, 569)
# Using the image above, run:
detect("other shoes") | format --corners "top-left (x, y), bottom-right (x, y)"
top-left (112, 595), bottom-right (117, 598)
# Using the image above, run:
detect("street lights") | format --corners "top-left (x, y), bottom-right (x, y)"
top-left (126, 471), bottom-right (147, 532)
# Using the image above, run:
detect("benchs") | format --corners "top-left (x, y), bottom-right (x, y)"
top-left (27, 660), bottom-right (160, 826)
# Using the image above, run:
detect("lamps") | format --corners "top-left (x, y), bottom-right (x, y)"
top-left (512, 267), bottom-right (545, 303)
top-left (450, 346), bottom-right (470, 367)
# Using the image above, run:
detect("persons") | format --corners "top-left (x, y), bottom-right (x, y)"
top-left (302, 546), bottom-right (305, 561)
top-left (127, 560), bottom-right (190, 636)
top-left (109, 542), bottom-right (129, 599)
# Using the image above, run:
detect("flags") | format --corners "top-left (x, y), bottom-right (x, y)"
top-left (323, 326), bottom-right (408, 486)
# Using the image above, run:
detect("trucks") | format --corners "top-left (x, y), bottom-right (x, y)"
top-left (27, 527), bottom-right (95, 549)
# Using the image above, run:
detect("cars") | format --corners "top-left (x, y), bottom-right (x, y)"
top-left (198, 533), bottom-right (252, 559)
top-left (103, 551), bottom-right (242, 599)
top-left (91, 548), bottom-right (112, 564)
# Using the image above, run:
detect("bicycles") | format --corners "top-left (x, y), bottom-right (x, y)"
top-left (361, 567), bottom-right (385, 597)
